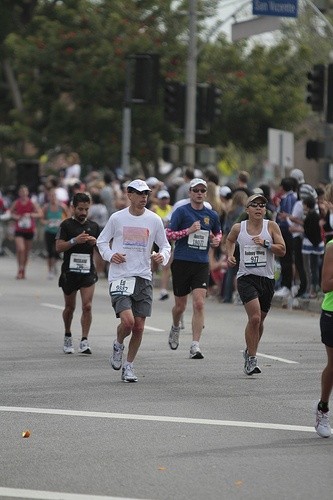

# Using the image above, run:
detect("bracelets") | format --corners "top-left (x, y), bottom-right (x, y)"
top-left (267, 243), bottom-right (271, 249)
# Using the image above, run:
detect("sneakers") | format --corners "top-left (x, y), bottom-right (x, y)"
top-left (241, 350), bottom-right (261, 377)
top-left (189, 345), bottom-right (204, 358)
top-left (111, 341), bottom-right (124, 370)
top-left (63, 336), bottom-right (75, 354)
top-left (78, 339), bottom-right (92, 354)
top-left (120, 363), bottom-right (138, 382)
top-left (314, 408), bottom-right (331, 437)
top-left (168, 325), bottom-right (181, 350)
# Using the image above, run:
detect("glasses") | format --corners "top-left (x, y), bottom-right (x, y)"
top-left (190, 188), bottom-right (207, 193)
top-left (132, 191), bottom-right (149, 195)
top-left (248, 202), bottom-right (266, 207)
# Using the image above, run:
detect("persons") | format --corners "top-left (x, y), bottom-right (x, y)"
top-left (227, 194), bottom-right (286, 375)
top-left (96, 179), bottom-right (171, 382)
top-left (315, 238), bottom-right (333, 437)
top-left (163, 180), bottom-right (222, 359)
top-left (56, 193), bottom-right (100, 352)
top-left (0, 152), bottom-right (333, 303)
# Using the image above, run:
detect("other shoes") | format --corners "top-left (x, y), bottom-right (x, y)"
top-left (296, 291), bottom-right (318, 301)
top-left (274, 286), bottom-right (291, 297)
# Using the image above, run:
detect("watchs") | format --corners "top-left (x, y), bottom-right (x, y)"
top-left (263, 240), bottom-right (270, 247)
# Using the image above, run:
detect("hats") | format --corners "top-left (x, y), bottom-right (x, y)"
top-left (157, 190), bottom-right (170, 200)
top-left (188, 178), bottom-right (208, 191)
top-left (127, 180), bottom-right (151, 192)
top-left (246, 194), bottom-right (269, 210)
top-left (289, 169), bottom-right (306, 185)
